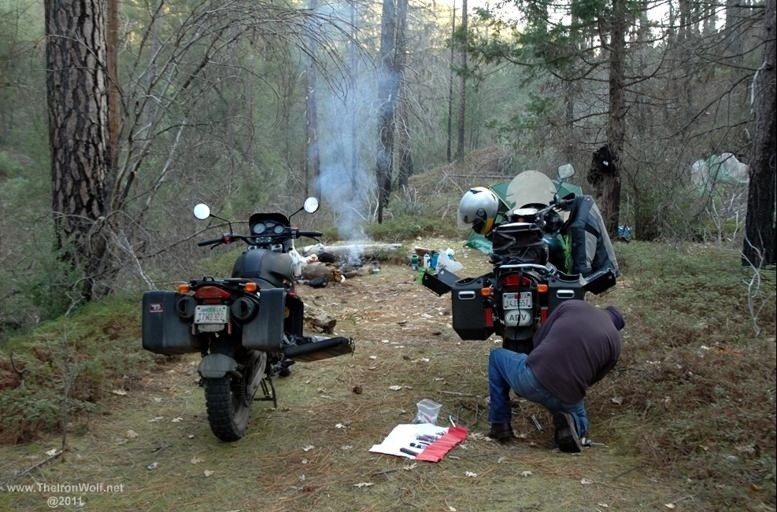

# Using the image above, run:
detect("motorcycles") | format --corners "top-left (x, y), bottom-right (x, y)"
top-left (421, 163), bottom-right (617, 356)
top-left (141, 195), bottom-right (354, 443)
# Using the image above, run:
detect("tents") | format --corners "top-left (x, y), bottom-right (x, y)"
top-left (463, 180), bottom-right (583, 254)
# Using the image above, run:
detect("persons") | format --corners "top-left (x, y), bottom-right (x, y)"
top-left (484, 300), bottom-right (626, 453)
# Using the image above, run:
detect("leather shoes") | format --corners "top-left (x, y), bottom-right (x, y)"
top-left (485, 422), bottom-right (512, 437)
top-left (555, 410), bottom-right (579, 452)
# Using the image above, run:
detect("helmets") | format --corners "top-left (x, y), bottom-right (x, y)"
top-left (459, 187), bottom-right (498, 237)
